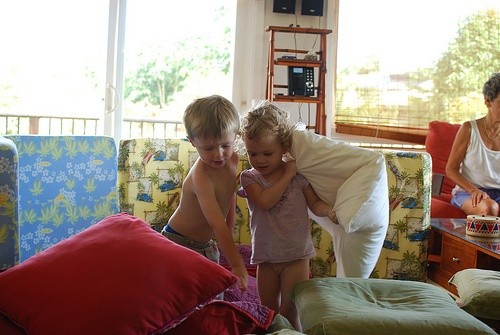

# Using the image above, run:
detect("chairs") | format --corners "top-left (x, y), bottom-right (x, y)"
top-left (425, 121), bottom-right (467, 218)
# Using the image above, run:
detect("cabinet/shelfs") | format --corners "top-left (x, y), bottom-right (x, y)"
top-left (264, 26), bottom-right (333, 136)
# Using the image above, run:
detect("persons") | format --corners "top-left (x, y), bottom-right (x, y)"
top-left (445, 72), bottom-right (500, 217)
top-left (160, 95), bottom-right (250, 292)
top-left (239, 103), bottom-right (340, 332)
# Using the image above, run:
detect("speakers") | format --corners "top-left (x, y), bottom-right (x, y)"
top-left (273, 0), bottom-right (296, 14)
top-left (301, 0), bottom-right (324, 16)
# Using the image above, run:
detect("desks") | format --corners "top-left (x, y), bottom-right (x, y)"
top-left (430, 218), bottom-right (500, 298)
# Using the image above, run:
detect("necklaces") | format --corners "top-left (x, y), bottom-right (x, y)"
top-left (484, 116), bottom-right (500, 141)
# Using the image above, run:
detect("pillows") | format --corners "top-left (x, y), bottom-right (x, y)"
top-left (295, 268), bottom-right (500, 335)
top-left (0, 211), bottom-right (239, 335)
